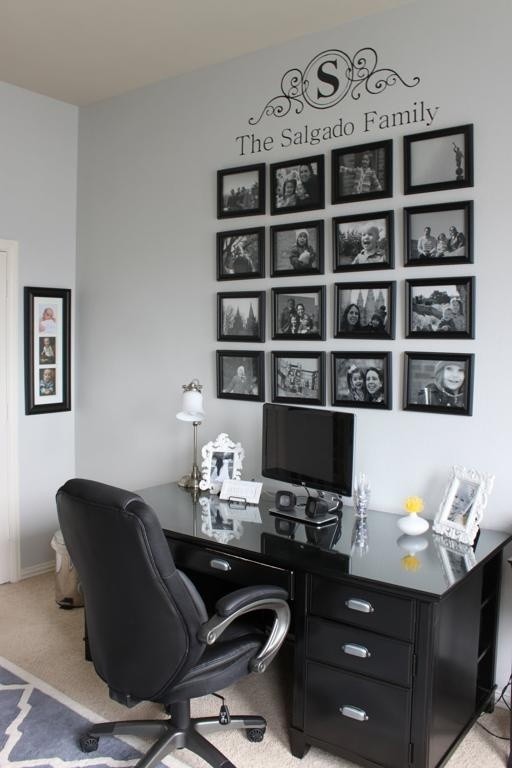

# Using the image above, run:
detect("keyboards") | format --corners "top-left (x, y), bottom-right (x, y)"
top-left (268, 505), bottom-right (338, 525)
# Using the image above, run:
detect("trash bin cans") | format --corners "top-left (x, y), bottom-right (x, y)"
top-left (49, 529), bottom-right (85, 610)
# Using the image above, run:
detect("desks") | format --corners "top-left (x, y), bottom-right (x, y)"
top-left (86, 482), bottom-right (512, 767)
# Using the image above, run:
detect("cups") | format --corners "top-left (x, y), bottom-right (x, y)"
top-left (353, 468), bottom-right (372, 519)
top-left (351, 518), bottom-right (369, 558)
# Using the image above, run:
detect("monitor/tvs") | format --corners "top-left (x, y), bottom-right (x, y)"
top-left (261, 403), bottom-right (355, 513)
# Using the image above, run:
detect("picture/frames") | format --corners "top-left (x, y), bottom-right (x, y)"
top-left (24, 287), bottom-right (71, 414)
top-left (432, 535), bottom-right (476, 586)
top-left (200, 497), bottom-right (243, 542)
top-left (198, 432), bottom-right (246, 494)
top-left (433, 466), bottom-right (493, 546)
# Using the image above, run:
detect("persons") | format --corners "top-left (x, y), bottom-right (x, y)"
top-left (435, 233), bottom-right (448, 258)
top-left (279, 297), bottom-right (388, 335)
top-left (213, 508), bottom-right (223, 528)
top-left (417, 225), bottom-right (437, 258)
top-left (443, 225), bottom-right (464, 256)
top-left (39, 305), bottom-right (55, 331)
top-left (40, 337), bottom-right (55, 365)
top-left (284, 369), bottom-right (304, 396)
top-left (215, 454), bottom-right (223, 477)
top-left (339, 151), bottom-right (383, 195)
top-left (445, 551), bottom-right (468, 581)
top-left (451, 142), bottom-right (463, 167)
top-left (344, 365), bottom-right (365, 402)
top-left (418, 361), bottom-right (466, 407)
top-left (447, 481), bottom-right (474, 526)
top-left (286, 229), bottom-right (317, 271)
top-left (232, 244), bottom-right (255, 272)
top-left (226, 163), bottom-right (318, 211)
top-left (219, 506), bottom-right (229, 527)
top-left (360, 365), bottom-right (383, 404)
top-left (219, 457), bottom-right (230, 479)
top-left (40, 368), bottom-right (55, 393)
top-left (448, 298), bottom-right (465, 332)
top-left (349, 227), bottom-right (388, 264)
top-left (434, 307), bottom-right (456, 332)
top-left (222, 364), bottom-right (253, 394)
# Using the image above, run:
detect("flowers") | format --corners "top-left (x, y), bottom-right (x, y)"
top-left (403, 556), bottom-right (419, 568)
top-left (404, 495), bottom-right (424, 513)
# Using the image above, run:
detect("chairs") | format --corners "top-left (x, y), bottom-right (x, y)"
top-left (57, 478), bottom-right (292, 768)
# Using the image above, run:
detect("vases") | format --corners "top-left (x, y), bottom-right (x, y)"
top-left (397, 534), bottom-right (429, 556)
top-left (397, 514), bottom-right (429, 536)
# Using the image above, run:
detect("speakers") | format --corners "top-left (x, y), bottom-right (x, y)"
top-left (276, 491), bottom-right (297, 511)
top-left (305, 496), bottom-right (329, 518)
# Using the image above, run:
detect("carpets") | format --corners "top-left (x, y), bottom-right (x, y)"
top-left (0, 656), bottom-right (192, 768)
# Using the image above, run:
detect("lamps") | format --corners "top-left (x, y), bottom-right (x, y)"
top-left (176, 379), bottom-right (206, 488)
